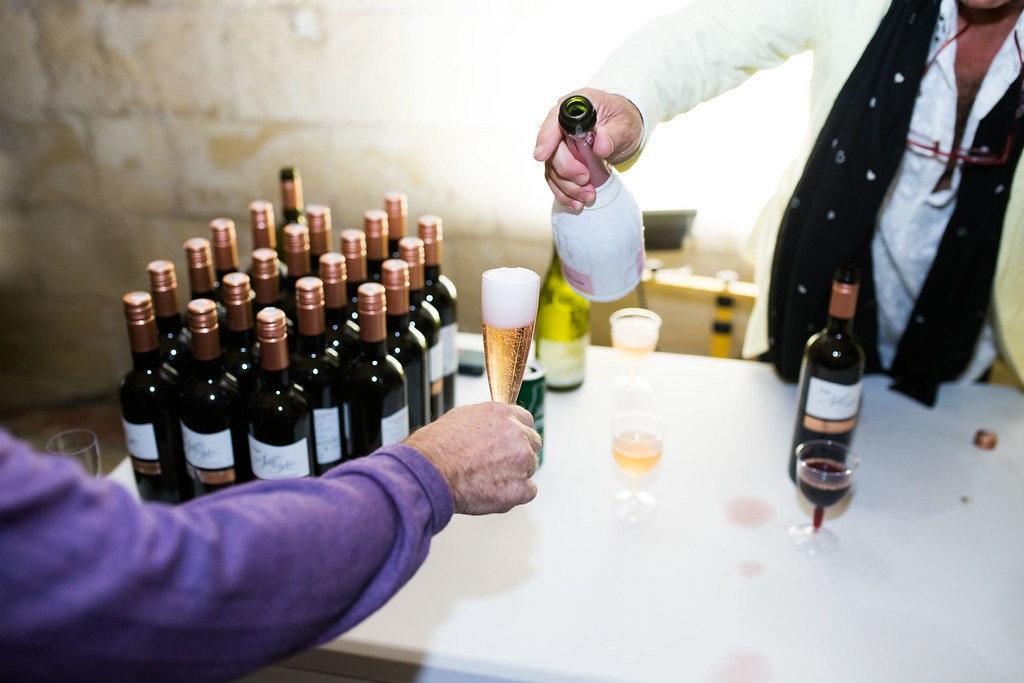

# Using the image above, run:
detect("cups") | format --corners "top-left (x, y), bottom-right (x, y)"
top-left (47, 427), bottom-right (102, 477)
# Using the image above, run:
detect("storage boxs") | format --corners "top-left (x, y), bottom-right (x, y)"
top-left (590, 249), bottom-right (757, 359)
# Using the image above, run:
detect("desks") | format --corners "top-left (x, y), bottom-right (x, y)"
top-left (105, 334), bottom-right (1023, 683)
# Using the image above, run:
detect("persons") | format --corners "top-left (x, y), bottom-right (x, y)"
top-left (0, 398), bottom-right (543, 682)
top-left (533, 0), bottom-right (1024, 408)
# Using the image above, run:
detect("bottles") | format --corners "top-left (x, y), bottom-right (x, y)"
top-left (550, 95), bottom-right (645, 303)
top-left (789, 272), bottom-right (866, 484)
top-left (535, 244), bottom-right (591, 392)
top-left (117, 166), bottom-right (462, 503)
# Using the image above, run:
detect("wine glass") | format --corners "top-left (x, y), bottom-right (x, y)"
top-left (610, 408), bottom-right (667, 518)
top-left (483, 267), bottom-right (540, 407)
top-left (607, 307), bottom-right (661, 405)
top-left (785, 439), bottom-right (860, 550)
